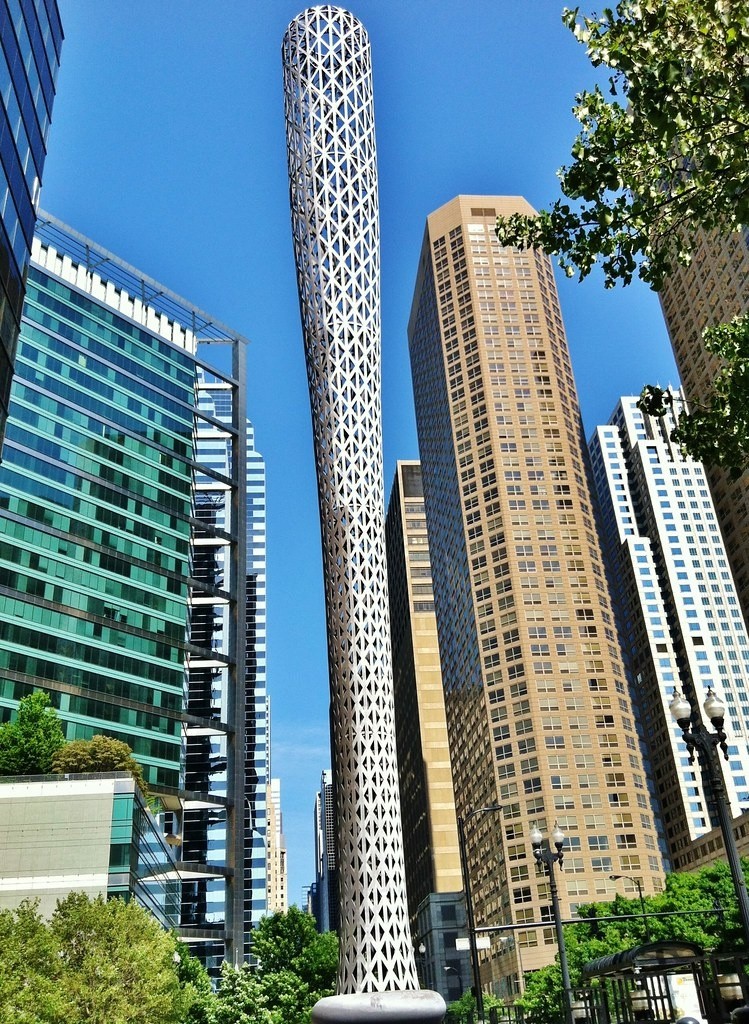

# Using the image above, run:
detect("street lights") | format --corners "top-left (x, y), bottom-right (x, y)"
top-left (444, 966), bottom-right (463, 994)
top-left (412, 944), bottom-right (426, 989)
top-left (528, 822), bottom-right (572, 990)
top-left (669, 684), bottom-right (749, 931)
top-left (458, 805), bottom-right (503, 1024)
top-left (610, 874), bottom-right (651, 942)
